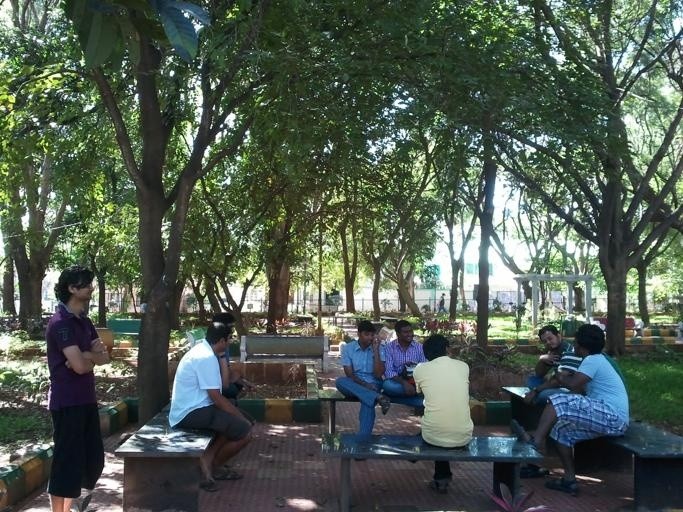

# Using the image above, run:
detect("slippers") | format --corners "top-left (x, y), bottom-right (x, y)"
top-left (200, 476), bottom-right (217, 491)
top-left (214, 465), bottom-right (243, 479)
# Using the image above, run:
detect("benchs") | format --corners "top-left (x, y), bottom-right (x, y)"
top-left (239, 336), bottom-right (330, 374)
top-left (333, 317), bottom-right (366, 326)
top-left (295, 314), bottom-right (313, 321)
top-left (318, 389), bottom-right (425, 434)
top-left (381, 316), bottom-right (398, 325)
top-left (320, 431), bottom-right (544, 511)
top-left (594, 318), bottom-right (635, 335)
top-left (114, 398), bottom-right (216, 512)
top-left (106, 318), bottom-right (141, 346)
top-left (186, 328), bottom-right (205, 347)
top-left (502, 386), bottom-right (683, 512)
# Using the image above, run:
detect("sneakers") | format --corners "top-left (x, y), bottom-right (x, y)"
top-left (519, 464), bottom-right (549, 478)
top-left (430, 480), bottom-right (448, 493)
top-left (378, 395), bottom-right (391, 415)
top-left (545, 477), bottom-right (577, 492)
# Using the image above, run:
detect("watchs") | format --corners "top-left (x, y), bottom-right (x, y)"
top-left (533, 387), bottom-right (539, 394)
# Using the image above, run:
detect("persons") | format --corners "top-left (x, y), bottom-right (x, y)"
top-left (381, 321), bottom-right (428, 408)
top-left (438, 293), bottom-right (448, 314)
top-left (336, 320), bottom-right (391, 462)
top-left (413, 334), bottom-right (475, 493)
top-left (212, 313), bottom-right (255, 467)
top-left (524, 325), bottom-right (583, 405)
top-left (169, 322), bottom-right (253, 492)
top-left (47, 267), bottom-right (111, 512)
top-left (511, 323), bottom-right (629, 494)
